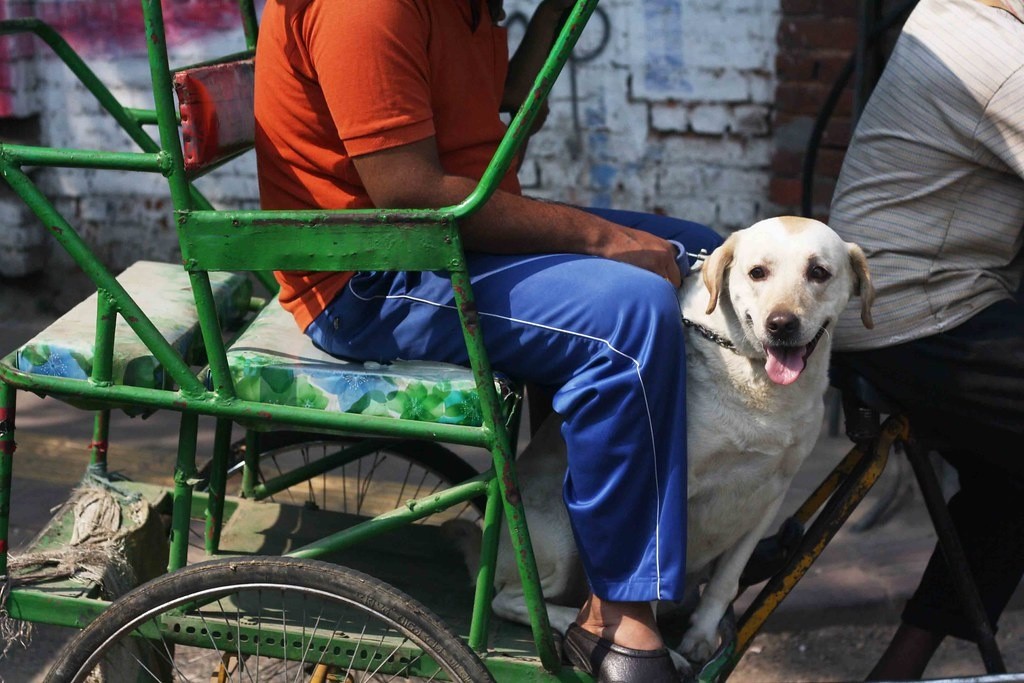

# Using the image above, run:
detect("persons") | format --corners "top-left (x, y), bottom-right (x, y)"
top-left (239, 2), bottom-right (695, 683)
top-left (815, 0), bottom-right (1024, 645)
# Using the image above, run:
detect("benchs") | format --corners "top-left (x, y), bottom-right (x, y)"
top-left (18, 260), bottom-right (253, 421)
top-left (195, 287), bottom-right (526, 431)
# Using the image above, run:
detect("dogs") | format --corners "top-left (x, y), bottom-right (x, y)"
top-left (446, 215), bottom-right (876, 683)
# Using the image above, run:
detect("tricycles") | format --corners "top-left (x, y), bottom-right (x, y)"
top-left (0, 0), bottom-right (1024, 682)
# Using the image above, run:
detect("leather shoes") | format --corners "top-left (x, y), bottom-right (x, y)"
top-left (566, 623), bottom-right (685, 683)
